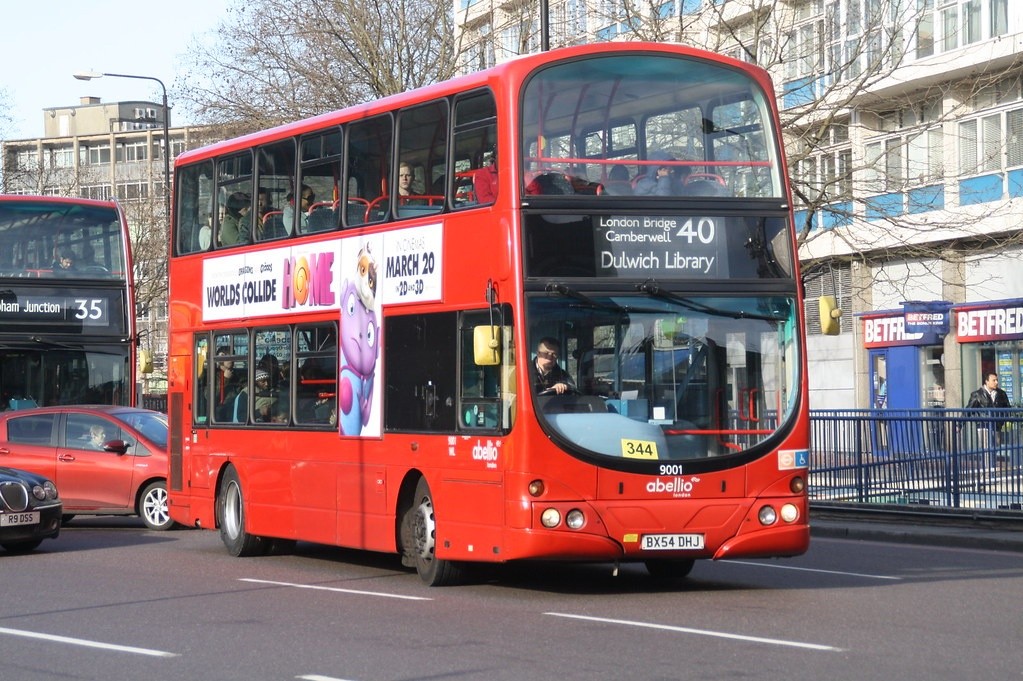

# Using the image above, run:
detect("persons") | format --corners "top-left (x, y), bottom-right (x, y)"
top-left (532, 337), bottom-right (575, 393)
top-left (203, 346), bottom-right (336, 423)
top-left (199, 203), bottom-right (226, 249)
top-left (378, 161), bottom-right (423, 221)
top-left (221, 192), bottom-right (252, 246)
top-left (87, 424), bottom-right (127, 449)
top-left (50, 250), bottom-right (78, 271)
top-left (474, 146), bottom-right (543, 204)
top-left (238, 187), bottom-right (282, 244)
top-left (610, 164), bottom-right (633, 192)
top-left (572, 167), bottom-right (590, 192)
top-left (956, 371), bottom-right (1011, 479)
top-left (283, 184), bottom-right (315, 234)
top-left (634, 151), bottom-right (686, 196)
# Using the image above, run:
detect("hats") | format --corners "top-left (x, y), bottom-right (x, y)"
top-left (255, 369), bottom-right (269, 382)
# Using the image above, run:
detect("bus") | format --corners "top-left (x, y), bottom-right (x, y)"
top-left (0, 194), bottom-right (153, 521)
top-left (166, 42), bottom-right (843, 588)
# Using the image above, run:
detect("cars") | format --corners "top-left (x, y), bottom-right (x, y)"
top-left (0, 464), bottom-right (62, 552)
top-left (0, 405), bottom-right (180, 531)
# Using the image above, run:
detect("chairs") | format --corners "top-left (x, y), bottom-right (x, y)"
top-left (263, 168), bottom-right (728, 239)
top-left (232, 390), bottom-right (248, 422)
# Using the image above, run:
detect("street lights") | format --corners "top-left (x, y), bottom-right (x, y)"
top-left (73, 69), bottom-right (170, 292)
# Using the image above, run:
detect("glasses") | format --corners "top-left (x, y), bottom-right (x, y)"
top-left (301, 194), bottom-right (315, 201)
